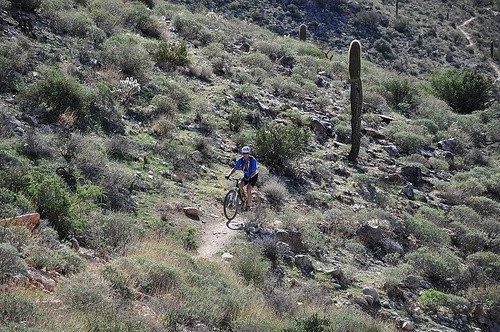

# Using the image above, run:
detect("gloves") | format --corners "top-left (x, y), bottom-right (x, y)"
top-left (225, 175), bottom-right (229, 179)
top-left (244, 179), bottom-right (249, 185)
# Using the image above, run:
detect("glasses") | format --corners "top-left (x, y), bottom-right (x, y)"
top-left (243, 153), bottom-right (249, 154)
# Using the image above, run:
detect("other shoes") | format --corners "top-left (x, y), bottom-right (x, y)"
top-left (246, 202), bottom-right (252, 208)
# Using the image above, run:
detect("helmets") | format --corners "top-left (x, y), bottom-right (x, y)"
top-left (241, 145), bottom-right (251, 154)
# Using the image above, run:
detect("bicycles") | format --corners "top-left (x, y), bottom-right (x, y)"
top-left (223, 175), bottom-right (262, 220)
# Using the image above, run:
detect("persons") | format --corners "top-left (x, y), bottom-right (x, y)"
top-left (226, 146), bottom-right (258, 208)
